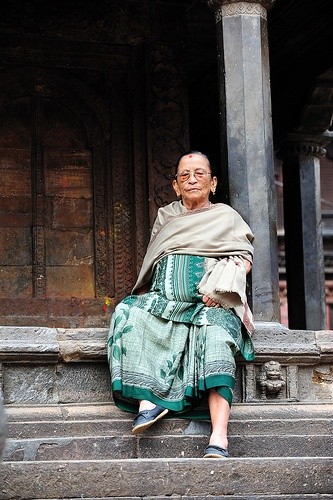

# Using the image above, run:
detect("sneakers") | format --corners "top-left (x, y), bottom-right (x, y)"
top-left (131, 403), bottom-right (169, 434)
top-left (202, 445), bottom-right (230, 459)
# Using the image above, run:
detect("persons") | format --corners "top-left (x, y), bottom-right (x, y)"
top-left (104, 149), bottom-right (256, 458)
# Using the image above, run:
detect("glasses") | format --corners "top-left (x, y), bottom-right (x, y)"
top-left (174, 168), bottom-right (212, 183)
